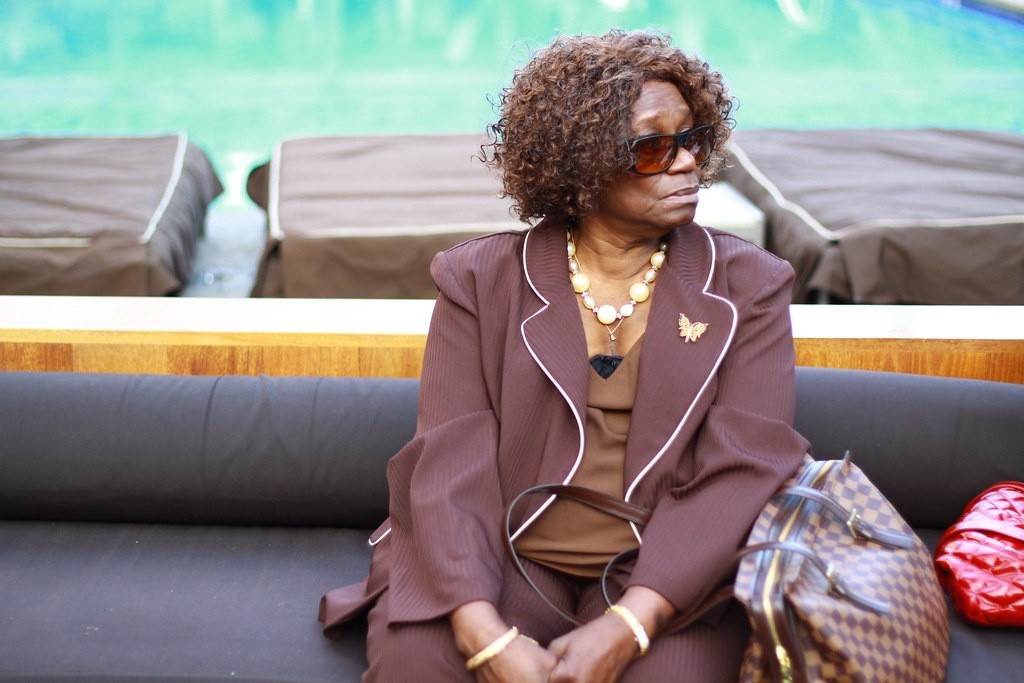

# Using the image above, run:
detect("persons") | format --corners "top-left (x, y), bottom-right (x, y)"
top-left (317, 25), bottom-right (813, 683)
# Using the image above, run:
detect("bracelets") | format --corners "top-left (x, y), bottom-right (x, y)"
top-left (605, 604), bottom-right (649, 655)
top-left (465, 625), bottom-right (518, 669)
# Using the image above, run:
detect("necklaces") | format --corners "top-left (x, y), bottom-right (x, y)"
top-left (566, 225), bottom-right (668, 341)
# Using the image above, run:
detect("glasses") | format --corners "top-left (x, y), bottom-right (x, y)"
top-left (620, 125), bottom-right (716, 175)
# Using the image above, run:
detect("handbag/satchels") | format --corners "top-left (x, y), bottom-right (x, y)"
top-left (934, 480), bottom-right (1024, 626)
top-left (503, 451), bottom-right (949, 683)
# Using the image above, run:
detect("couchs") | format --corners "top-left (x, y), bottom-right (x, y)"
top-left (0, 369), bottom-right (1024, 683)
